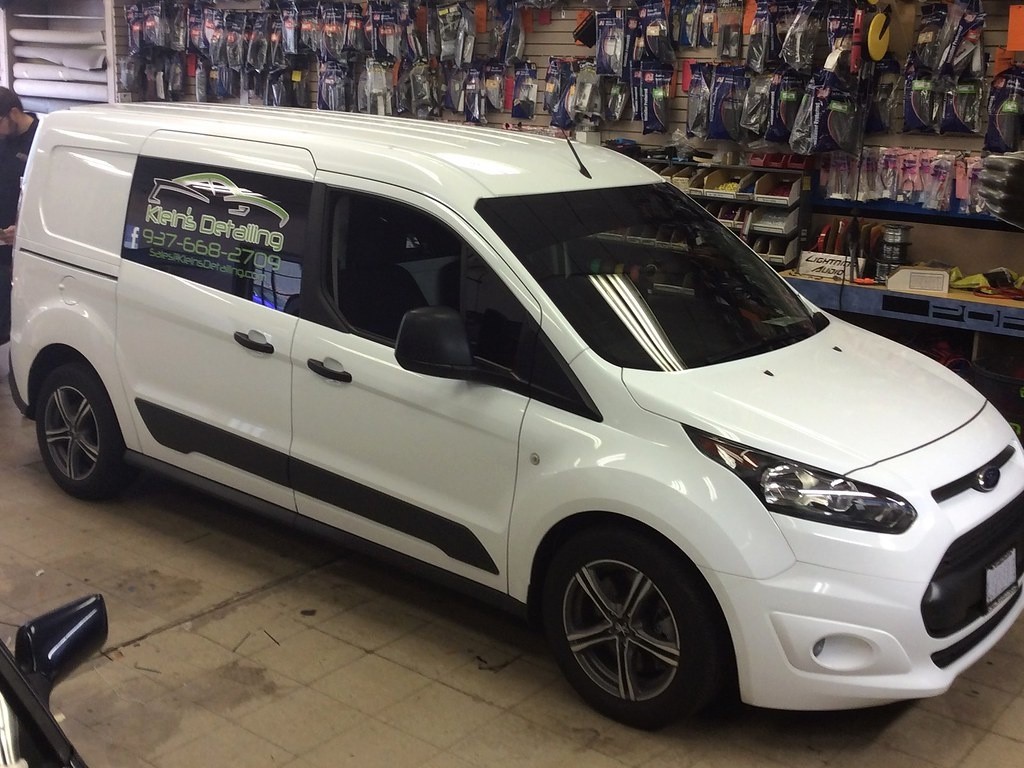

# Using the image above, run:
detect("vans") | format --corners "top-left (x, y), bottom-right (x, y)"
top-left (8, 98), bottom-right (1024, 733)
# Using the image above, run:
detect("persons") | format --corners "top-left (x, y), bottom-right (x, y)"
top-left (0, 85), bottom-right (38, 380)
